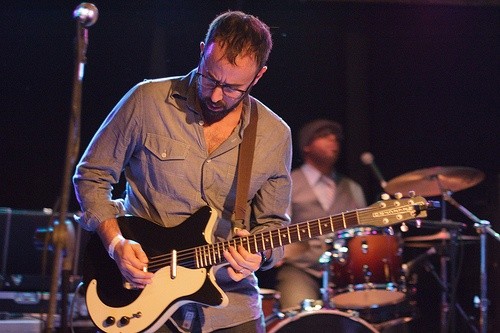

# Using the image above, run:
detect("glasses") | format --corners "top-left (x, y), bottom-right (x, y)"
top-left (196, 52), bottom-right (264, 99)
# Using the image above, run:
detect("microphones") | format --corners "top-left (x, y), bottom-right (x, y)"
top-left (402, 249), bottom-right (437, 271)
top-left (361, 152), bottom-right (387, 189)
top-left (73, 2), bottom-right (98, 27)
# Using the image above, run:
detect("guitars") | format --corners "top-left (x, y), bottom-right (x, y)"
top-left (83, 190), bottom-right (441, 333)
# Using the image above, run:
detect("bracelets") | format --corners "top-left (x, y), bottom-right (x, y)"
top-left (108, 235), bottom-right (123, 260)
top-left (261, 252), bottom-right (267, 264)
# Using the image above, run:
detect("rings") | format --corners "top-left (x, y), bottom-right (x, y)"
top-left (239, 269), bottom-right (244, 272)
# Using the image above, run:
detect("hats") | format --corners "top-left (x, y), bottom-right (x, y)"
top-left (299, 120), bottom-right (341, 147)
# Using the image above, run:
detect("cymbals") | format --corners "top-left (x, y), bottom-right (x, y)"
top-left (404, 231), bottom-right (481, 248)
top-left (385, 166), bottom-right (485, 196)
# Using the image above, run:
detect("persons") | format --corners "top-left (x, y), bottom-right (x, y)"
top-left (72, 10), bottom-right (293, 333)
top-left (272, 118), bottom-right (367, 311)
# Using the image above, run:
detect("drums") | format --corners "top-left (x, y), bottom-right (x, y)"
top-left (326, 223), bottom-right (412, 308)
top-left (268, 308), bottom-right (380, 333)
top-left (260, 288), bottom-right (281, 319)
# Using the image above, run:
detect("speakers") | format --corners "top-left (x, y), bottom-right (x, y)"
top-left (0, 207), bottom-right (89, 293)
